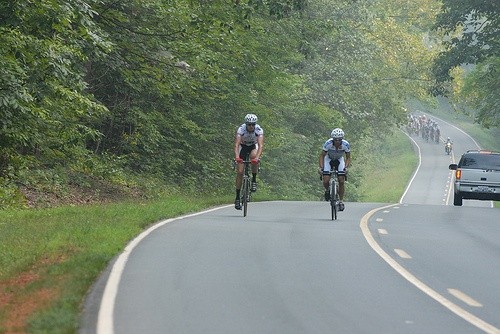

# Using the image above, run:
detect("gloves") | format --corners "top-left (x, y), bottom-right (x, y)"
top-left (318, 168), bottom-right (323, 175)
top-left (252, 158), bottom-right (259, 164)
top-left (343, 167), bottom-right (349, 174)
top-left (236, 158), bottom-right (243, 164)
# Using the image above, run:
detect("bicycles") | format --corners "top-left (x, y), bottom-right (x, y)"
top-left (234, 157), bottom-right (263, 217)
top-left (320, 168), bottom-right (350, 220)
top-left (402, 107), bottom-right (454, 154)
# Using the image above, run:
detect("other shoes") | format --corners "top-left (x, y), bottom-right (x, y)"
top-left (235, 198), bottom-right (241, 210)
top-left (325, 189), bottom-right (330, 201)
top-left (339, 201), bottom-right (344, 211)
top-left (252, 181), bottom-right (257, 192)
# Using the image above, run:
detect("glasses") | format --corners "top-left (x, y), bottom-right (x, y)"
top-left (334, 138), bottom-right (342, 141)
top-left (246, 123), bottom-right (255, 125)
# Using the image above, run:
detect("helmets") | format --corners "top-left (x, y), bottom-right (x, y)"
top-left (244, 113), bottom-right (258, 123)
top-left (330, 127), bottom-right (344, 137)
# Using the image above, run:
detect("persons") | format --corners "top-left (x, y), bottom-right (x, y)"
top-left (234, 114), bottom-right (263, 209)
top-left (403, 114), bottom-right (440, 140)
top-left (320, 128), bottom-right (351, 211)
top-left (444, 137), bottom-right (454, 152)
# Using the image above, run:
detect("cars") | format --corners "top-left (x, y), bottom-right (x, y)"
top-left (449, 150), bottom-right (498, 205)
